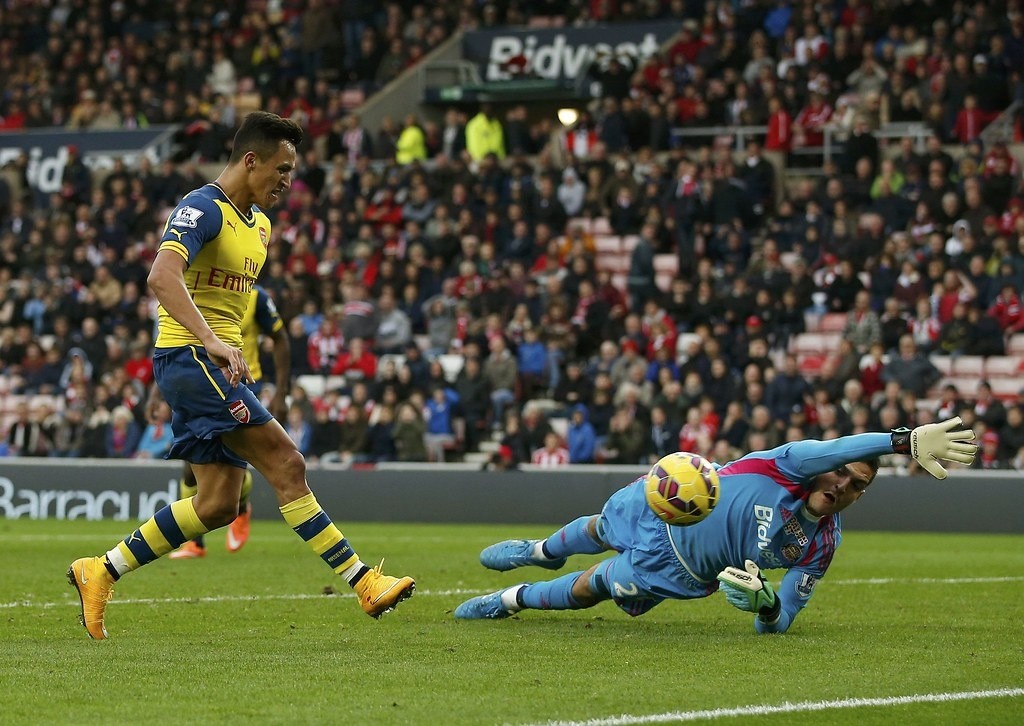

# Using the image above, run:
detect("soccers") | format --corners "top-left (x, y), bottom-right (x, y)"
top-left (642, 450), bottom-right (721, 529)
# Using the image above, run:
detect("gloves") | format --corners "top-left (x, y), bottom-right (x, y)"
top-left (890, 416), bottom-right (978, 481)
top-left (716, 559), bottom-right (782, 627)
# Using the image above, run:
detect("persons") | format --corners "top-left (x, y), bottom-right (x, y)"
top-left (455, 416), bottom-right (978, 636)
top-left (0, 0), bottom-right (1024, 560)
top-left (65, 112), bottom-right (415, 638)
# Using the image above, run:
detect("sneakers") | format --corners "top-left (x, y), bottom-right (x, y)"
top-left (355, 557), bottom-right (417, 620)
top-left (225, 501), bottom-right (252, 553)
top-left (66, 555), bottom-right (118, 641)
top-left (168, 540), bottom-right (208, 559)
top-left (479, 538), bottom-right (567, 572)
top-left (454, 581), bottom-right (536, 620)
